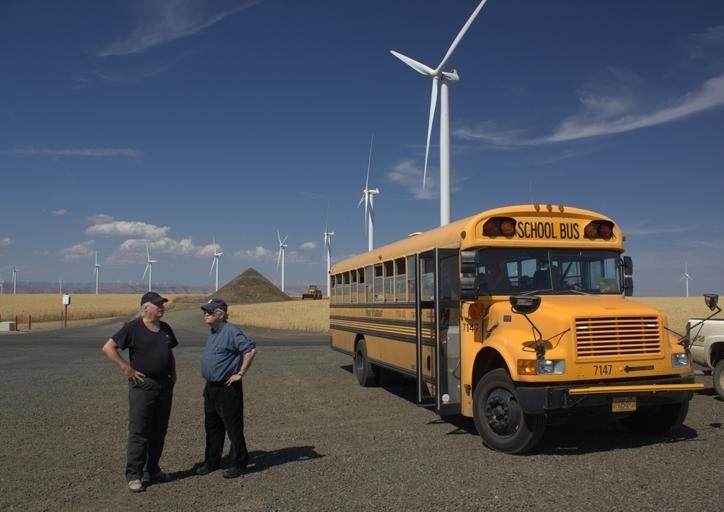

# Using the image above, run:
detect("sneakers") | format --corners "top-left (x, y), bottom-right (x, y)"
top-left (143, 470), bottom-right (172, 483)
top-left (222, 460), bottom-right (247, 478)
top-left (127, 479), bottom-right (144, 492)
top-left (192, 457), bottom-right (223, 475)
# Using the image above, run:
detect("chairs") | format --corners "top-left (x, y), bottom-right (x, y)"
top-left (530, 266), bottom-right (565, 295)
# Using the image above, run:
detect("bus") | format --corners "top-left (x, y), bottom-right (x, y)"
top-left (329, 203), bottom-right (722, 455)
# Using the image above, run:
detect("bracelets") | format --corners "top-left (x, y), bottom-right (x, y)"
top-left (237, 369), bottom-right (246, 377)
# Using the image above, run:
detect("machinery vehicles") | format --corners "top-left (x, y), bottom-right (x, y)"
top-left (302, 284), bottom-right (322, 300)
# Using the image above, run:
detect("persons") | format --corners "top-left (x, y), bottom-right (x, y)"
top-left (101, 291), bottom-right (178, 492)
top-left (190, 296), bottom-right (257, 478)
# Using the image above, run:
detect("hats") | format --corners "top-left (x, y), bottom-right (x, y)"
top-left (201, 299), bottom-right (228, 313)
top-left (141, 291), bottom-right (168, 306)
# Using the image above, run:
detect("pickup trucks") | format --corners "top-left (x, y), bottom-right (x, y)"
top-left (686, 317), bottom-right (724, 399)
